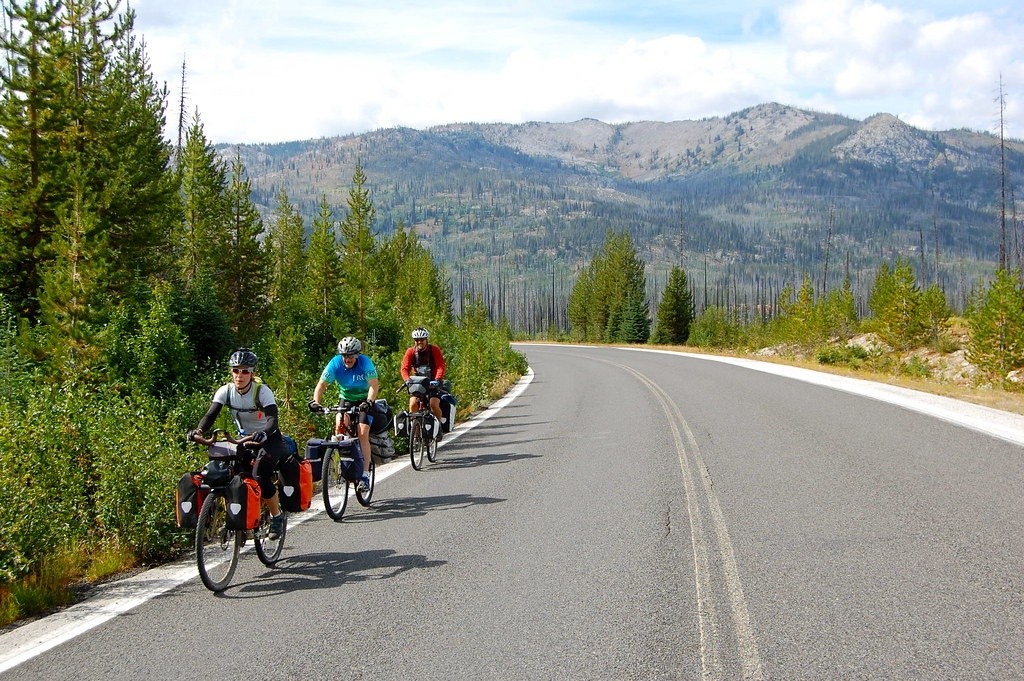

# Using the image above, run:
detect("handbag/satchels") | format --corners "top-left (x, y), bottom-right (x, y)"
top-left (175, 472), bottom-right (210, 528)
top-left (394, 409), bottom-right (409, 437)
top-left (338, 438), bottom-right (364, 480)
top-left (441, 400), bottom-right (457, 433)
top-left (421, 411), bottom-right (440, 439)
top-left (304, 437), bottom-right (326, 482)
top-left (277, 459), bottom-right (312, 512)
top-left (226, 472), bottom-right (262, 531)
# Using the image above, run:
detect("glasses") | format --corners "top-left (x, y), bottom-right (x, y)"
top-left (414, 338), bottom-right (425, 341)
top-left (343, 354), bottom-right (354, 358)
top-left (231, 368), bottom-right (251, 374)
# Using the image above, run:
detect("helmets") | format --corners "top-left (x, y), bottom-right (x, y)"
top-left (229, 351), bottom-right (258, 367)
top-left (411, 328), bottom-right (429, 338)
top-left (338, 336), bottom-right (361, 354)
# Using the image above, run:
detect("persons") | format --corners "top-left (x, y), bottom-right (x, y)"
top-left (187, 352), bottom-right (287, 541)
top-left (313, 336), bottom-right (379, 492)
top-left (401, 327), bottom-right (446, 442)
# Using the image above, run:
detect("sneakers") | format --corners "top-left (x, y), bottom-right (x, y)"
top-left (357, 476), bottom-right (370, 492)
top-left (269, 510), bottom-right (285, 540)
top-left (217, 523), bottom-right (227, 537)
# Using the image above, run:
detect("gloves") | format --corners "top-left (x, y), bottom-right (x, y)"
top-left (310, 402), bottom-right (319, 412)
top-left (405, 380), bottom-right (410, 387)
top-left (430, 379), bottom-right (440, 387)
top-left (187, 429), bottom-right (203, 441)
top-left (252, 430), bottom-right (268, 443)
top-left (359, 399), bottom-right (372, 413)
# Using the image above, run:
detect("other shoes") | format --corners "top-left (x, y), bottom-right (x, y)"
top-left (413, 444), bottom-right (418, 450)
top-left (437, 422), bottom-right (443, 442)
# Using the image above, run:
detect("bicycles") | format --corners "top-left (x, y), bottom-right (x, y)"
top-left (305, 398), bottom-right (393, 521)
top-left (393, 376), bottom-right (457, 471)
top-left (176, 428), bottom-right (313, 592)
